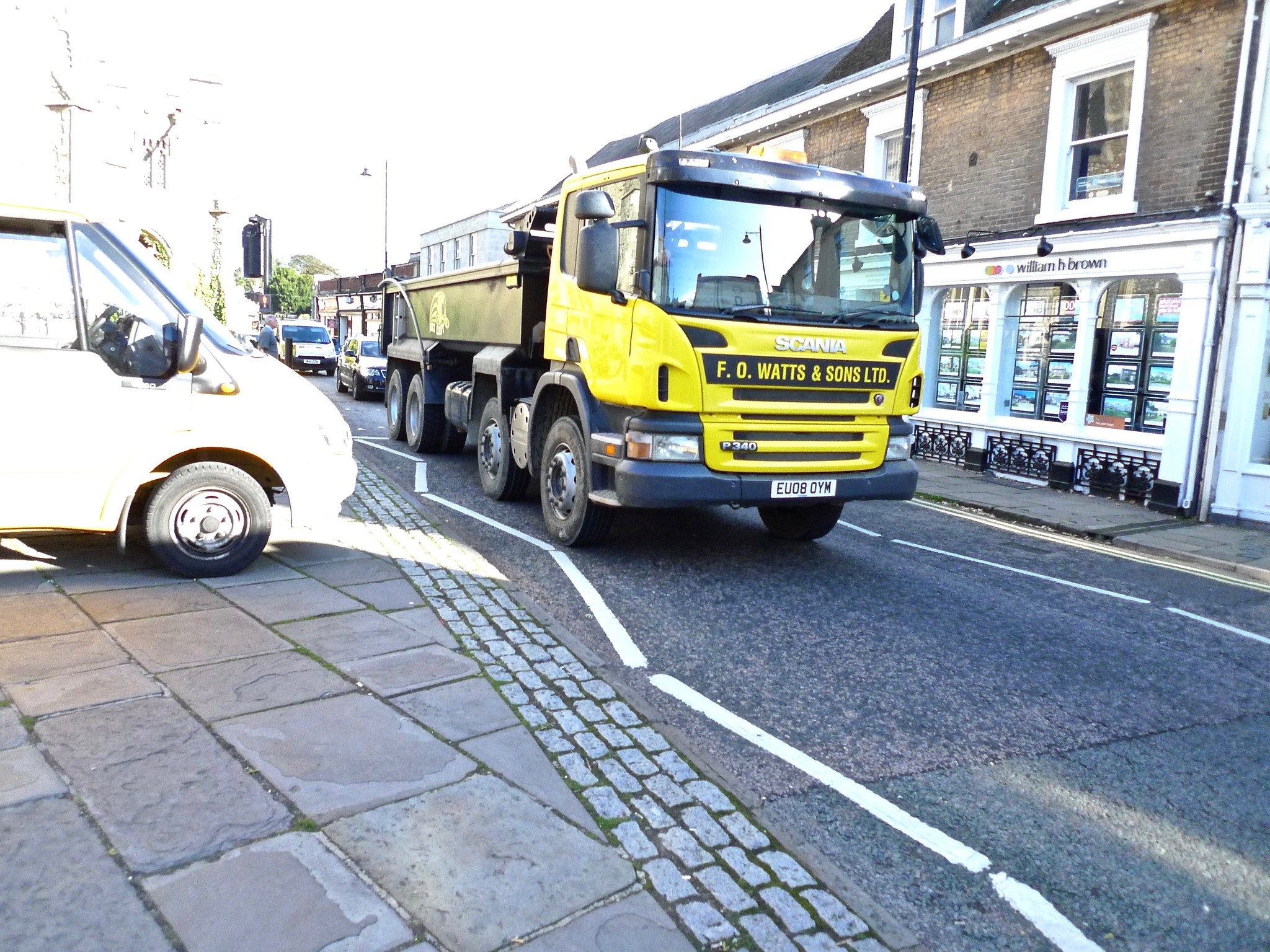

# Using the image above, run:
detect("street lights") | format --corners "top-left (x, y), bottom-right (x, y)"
top-left (362, 150), bottom-right (391, 284)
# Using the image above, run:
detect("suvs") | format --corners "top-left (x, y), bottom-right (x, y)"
top-left (274, 317), bottom-right (338, 377)
top-left (336, 333), bottom-right (388, 400)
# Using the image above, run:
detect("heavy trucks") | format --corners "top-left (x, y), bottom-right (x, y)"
top-left (377, 133), bottom-right (948, 552)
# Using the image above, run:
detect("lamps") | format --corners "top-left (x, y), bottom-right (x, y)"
top-left (852, 247), bottom-right (866, 273)
top-left (1036, 221), bottom-right (1081, 258)
top-left (960, 229), bottom-right (998, 259)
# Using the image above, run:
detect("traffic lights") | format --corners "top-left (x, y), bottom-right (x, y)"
top-left (258, 294), bottom-right (275, 314)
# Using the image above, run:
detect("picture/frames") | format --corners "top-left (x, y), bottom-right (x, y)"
top-left (1008, 281), bottom-right (1079, 424)
top-left (1099, 292), bottom-right (1182, 433)
top-left (934, 298), bottom-right (988, 414)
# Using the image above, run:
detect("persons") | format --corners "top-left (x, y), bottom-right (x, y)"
top-left (258, 315), bottom-right (278, 360)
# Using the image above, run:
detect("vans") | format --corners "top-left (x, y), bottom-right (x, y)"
top-left (0, 198), bottom-right (357, 582)
